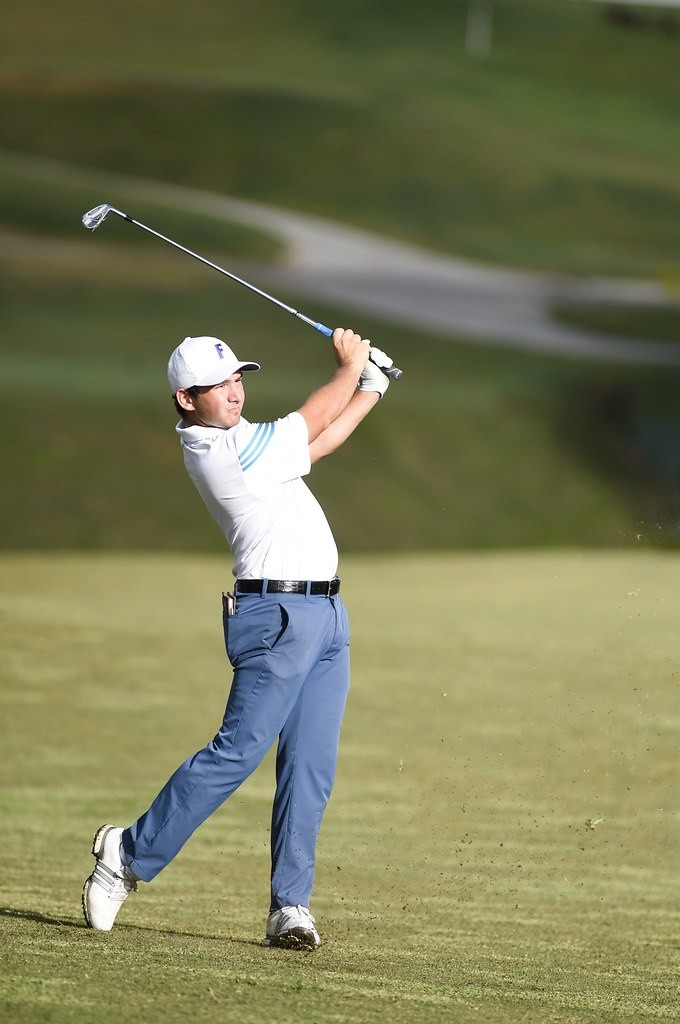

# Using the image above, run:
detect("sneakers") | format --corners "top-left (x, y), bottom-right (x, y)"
top-left (266, 904), bottom-right (319, 950)
top-left (81, 824), bottom-right (138, 932)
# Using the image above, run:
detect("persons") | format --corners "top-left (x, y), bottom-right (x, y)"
top-left (82, 328), bottom-right (394, 952)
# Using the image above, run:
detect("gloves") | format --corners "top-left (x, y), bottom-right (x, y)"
top-left (358, 339), bottom-right (394, 399)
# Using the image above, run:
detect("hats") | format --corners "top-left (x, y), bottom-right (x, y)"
top-left (167, 336), bottom-right (261, 394)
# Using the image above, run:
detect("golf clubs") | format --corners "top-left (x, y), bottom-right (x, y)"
top-left (81, 203), bottom-right (404, 381)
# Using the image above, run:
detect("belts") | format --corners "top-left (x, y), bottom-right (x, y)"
top-left (237, 578), bottom-right (341, 595)
